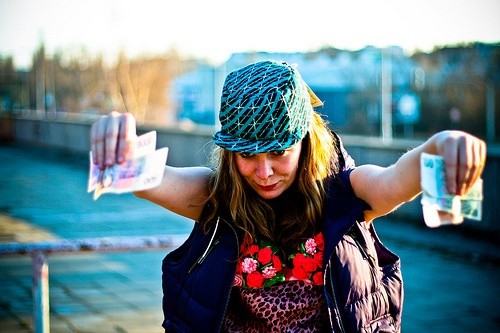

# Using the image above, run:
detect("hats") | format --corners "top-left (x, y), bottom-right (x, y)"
top-left (212, 61), bottom-right (313, 153)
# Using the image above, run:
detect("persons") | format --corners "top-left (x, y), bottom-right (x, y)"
top-left (90, 61), bottom-right (487, 333)
